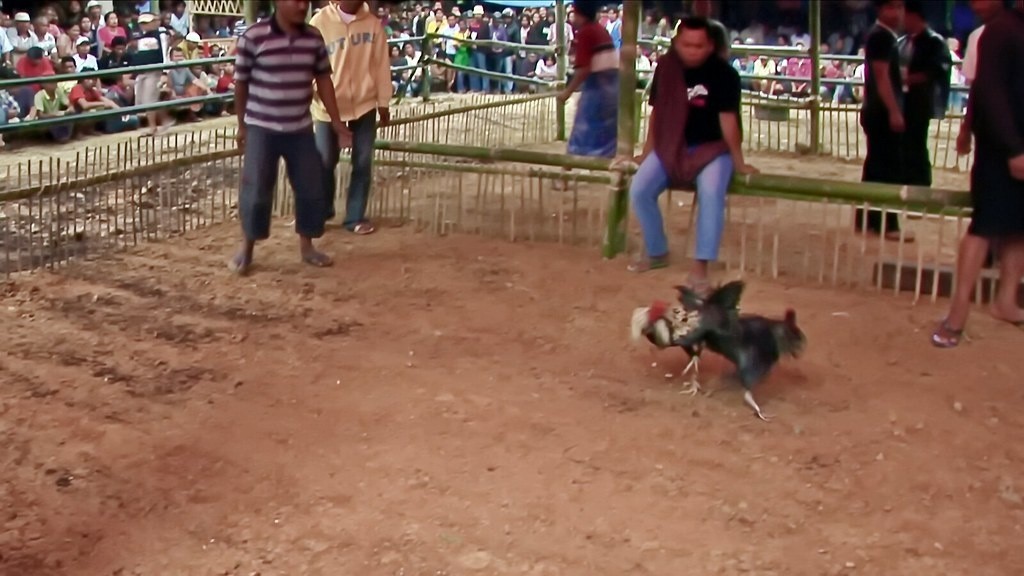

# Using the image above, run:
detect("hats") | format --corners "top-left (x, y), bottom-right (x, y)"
top-left (84, 0), bottom-right (102, 14)
top-left (473, 5), bottom-right (485, 15)
top-left (14, 12), bottom-right (30, 22)
top-left (186, 31), bottom-right (203, 43)
top-left (137, 14), bottom-right (159, 23)
top-left (76, 37), bottom-right (90, 46)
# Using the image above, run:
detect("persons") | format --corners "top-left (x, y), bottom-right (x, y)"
top-left (373, 1), bottom-right (1022, 156)
top-left (895, 1), bottom-right (953, 220)
top-left (307, 0), bottom-right (393, 234)
top-left (1, 0), bottom-right (266, 150)
top-left (625, 13), bottom-right (764, 284)
top-left (934, 0), bottom-right (1024, 345)
top-left (855, 0), bottom-right (918, 246)
top-left (234, 0), bottom-right (354, 280)
top-left (554, 2), bottom-right (620, 193)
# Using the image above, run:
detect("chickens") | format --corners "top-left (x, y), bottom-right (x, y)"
top-left (632, 279), bottom-right (806, 423)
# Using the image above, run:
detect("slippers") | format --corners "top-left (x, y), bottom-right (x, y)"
top-left (140, 118), bottom-right (177, 137)
top-left (932, 317), bottom-right (964, 347)
top-left (354, 224), bottom-right (374, 234)
